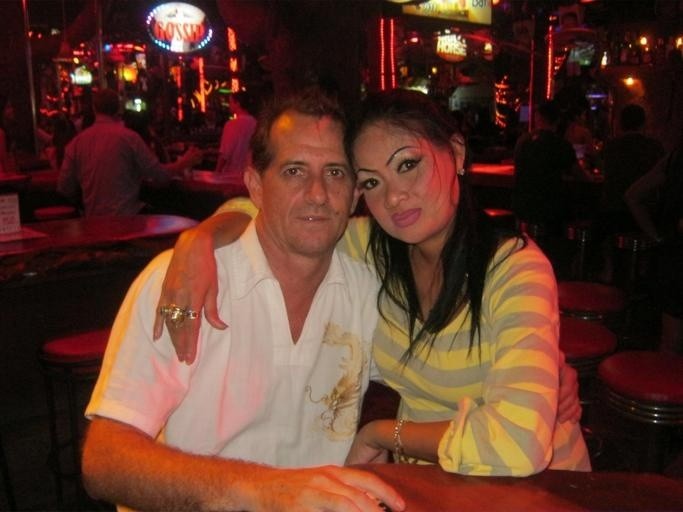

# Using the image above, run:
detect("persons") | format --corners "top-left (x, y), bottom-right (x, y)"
top-left (36, 116), bottom-right (56, 157)
top-left (56, 89), bottom-right (202, 220)
top-left (154, 87), bottom-right (593, 477)
top-left (216, 90), bottom-right (258, 177)
top-left (509, 83), bottom-right (683, 361)
top-left (80, 84), bottom-right (582, 509)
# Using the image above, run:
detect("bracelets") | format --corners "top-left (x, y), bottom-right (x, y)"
top-left (393, 418), bottom-right (419, 464)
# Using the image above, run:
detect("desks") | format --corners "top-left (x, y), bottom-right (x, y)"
top-left (344, 458), bottom-right (683, 510)
top-left (462, 163), bottom-right (516, 190)
top-left (144, 165), bottom-right (248, 215)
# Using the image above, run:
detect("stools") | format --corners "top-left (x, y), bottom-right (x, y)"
top-left (519, 219), bottom-right (555, 244)
top-left (559, 314), bottom-right (617, 368)
top-left (595, 348), bottom-right (680, 459)
top-left (565, 224), bottom-right (602, 279)
top-left (482, 207), bottom-right (513, 229)
top-left (38, 329), bottom-right (121, 511)
top-left (612, 229), bottom-right (659, 292)
top-left (556, 280), bottom-right (628, 315)
top-left (33, 204), bottom-right (77, 221)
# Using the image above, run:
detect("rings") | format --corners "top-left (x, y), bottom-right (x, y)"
top-left (158, 307), bottom-right (173, 314)
top-left (185, 311), bottom-right (200, 319)
top-left (169, 305), bottom-right (187, 329)
